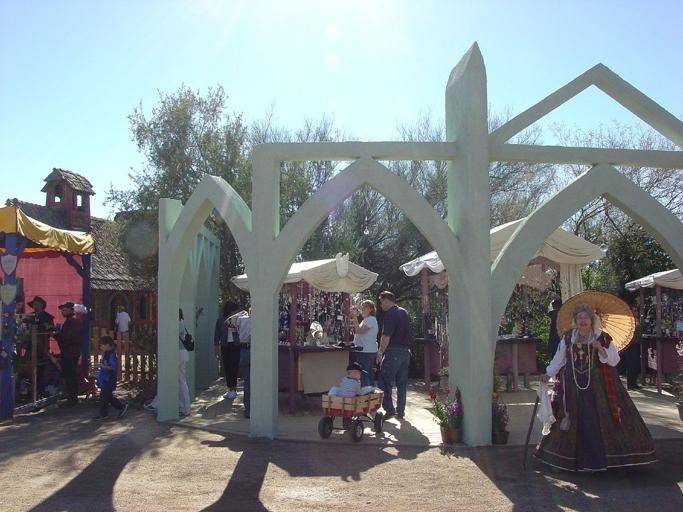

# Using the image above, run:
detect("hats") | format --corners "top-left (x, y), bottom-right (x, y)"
top-left (347, 361), bottom-right (369, 375)
top-left (28, 297), bottom-right (46, 309)
top-left (59, 302), bottom-right (74, 310)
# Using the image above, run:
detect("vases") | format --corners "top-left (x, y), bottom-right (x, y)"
top-left (493, 431), bottom-right (510, 444)
top-left (676, 404), bottom-right (683, 421)
top-left (440, 427), bottom-right (462, 444)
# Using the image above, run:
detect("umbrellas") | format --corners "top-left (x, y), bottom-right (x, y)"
top-left (557, 290), bottom-right (637, 354)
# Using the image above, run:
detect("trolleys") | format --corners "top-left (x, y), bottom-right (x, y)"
top-left (316, 362), bottom-right (383, 442)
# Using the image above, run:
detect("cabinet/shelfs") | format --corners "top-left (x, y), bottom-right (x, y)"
top-left (14, 330), bottom-right (58, 402)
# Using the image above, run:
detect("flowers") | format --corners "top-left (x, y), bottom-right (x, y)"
top-left (672, 341), bottom-right (683, 403)
top-left (429, 385), bottom-right (463, 428)
top-left (492, 399), bottom-right (510, 431)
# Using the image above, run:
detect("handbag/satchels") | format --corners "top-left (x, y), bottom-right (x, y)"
top-left (183, 334), bottom-right (194, 351)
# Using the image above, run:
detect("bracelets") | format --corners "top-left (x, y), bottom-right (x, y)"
top-left (378, 351), bottom-right (383, 356)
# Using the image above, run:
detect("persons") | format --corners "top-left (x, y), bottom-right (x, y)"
top-left (144, 308), bottom-right (191, 415)
top-left (533, 298), bottom-right (661, 472)
top-left (336, 362), bottom-right (376, 396)
top-left (93, 304), bottom-right (132, 420)
top-left (349, 300), bottom-right (379, 387)
top-left (625, 305), bottom-right (642, 391)
top-left (547, 298), bottom-right (562, 359)
top-left (22, 295), bottom-right (81, 409)
top-left (375, 290), bottom-right (411, 419)
top-left (320, 313), bottom-right (332, 327)
top-left (214, 300), bottom-right (241, 399)
top-left (223, 306), bottom-right (250, 419)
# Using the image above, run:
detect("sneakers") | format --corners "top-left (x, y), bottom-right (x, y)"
top-left (383, 413), bottom-right (394, 419)
top-left (118, 402), bottom-right (129, 418)
top-left (144, 401), bottom-right (157, 410)
top-left (223, 390), bottom-right (237, 399)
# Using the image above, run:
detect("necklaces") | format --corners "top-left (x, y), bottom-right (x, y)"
top-left (571, 329), bottom-right (591, 390)
